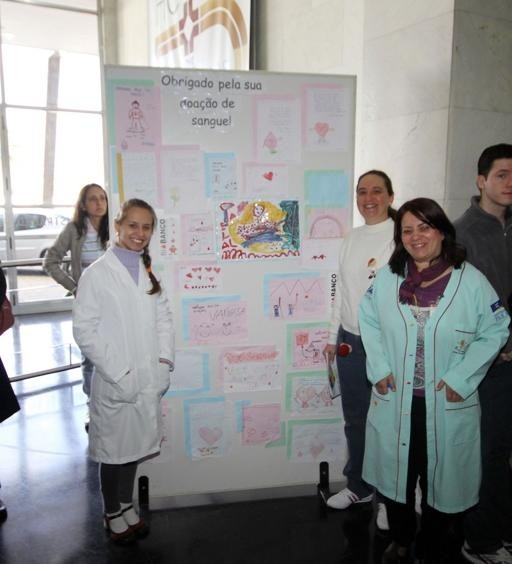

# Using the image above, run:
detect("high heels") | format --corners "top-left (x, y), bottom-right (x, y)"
top-left (105, 505), bottom-right (149, 545)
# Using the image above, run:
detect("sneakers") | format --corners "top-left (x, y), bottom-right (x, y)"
top-left (325, 488), bottom-right (372, 510)
top-left (376, 504), bottom-right (391, 530)
top-left (460, 540), bottom-right (512, 564)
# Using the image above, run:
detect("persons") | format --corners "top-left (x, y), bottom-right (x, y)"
top-left (0, 268), bottom-right (20, 522)
top-left (42, 183), bottom-right (110, 427)
top-left (321, 169), bottom-right (398, 531)
top-left (452, 142), bottom-right (512, 564)
top-left (72, 199), bottom-right (175, 543)
top-left (358, 198), bottom-right (511, 564)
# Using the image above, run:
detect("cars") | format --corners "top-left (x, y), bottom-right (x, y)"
top-left (0, 207), bottom-right (74, 276)
